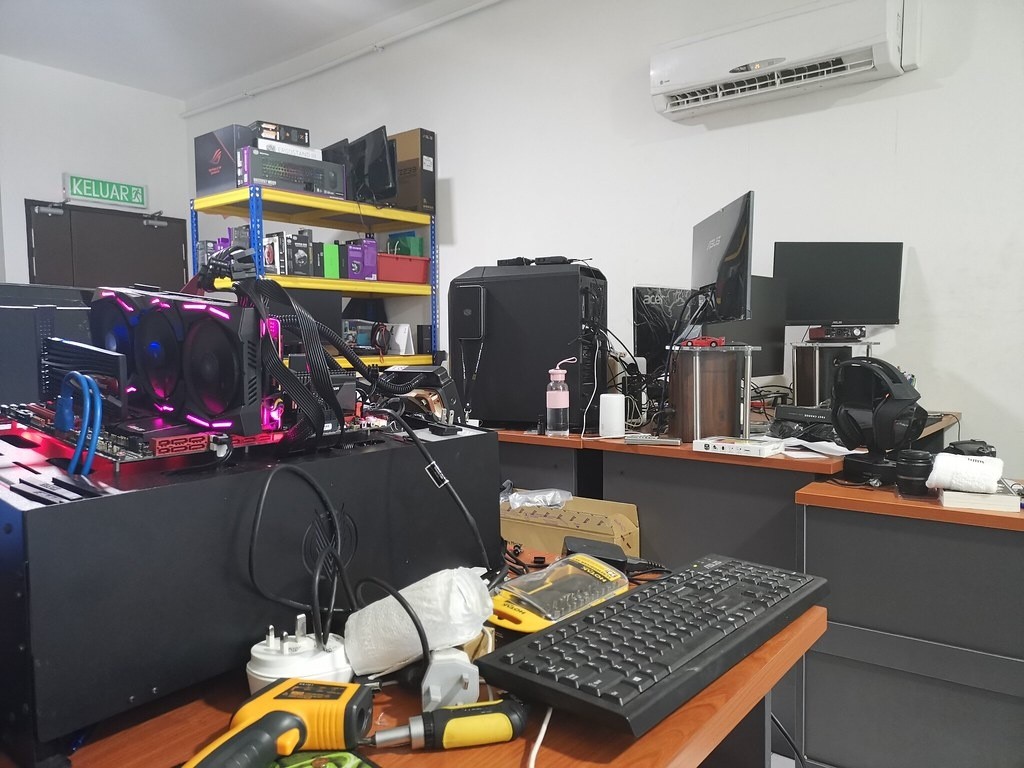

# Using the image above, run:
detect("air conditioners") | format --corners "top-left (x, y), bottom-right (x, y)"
top-left (648, 0), bottom-right (923, 125)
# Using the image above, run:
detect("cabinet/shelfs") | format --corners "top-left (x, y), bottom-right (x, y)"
top-left (189, 187), bottom-right (443, 389)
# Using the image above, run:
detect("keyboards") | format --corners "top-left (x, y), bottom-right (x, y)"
top-left (474, 553), bottom-right (831, 737)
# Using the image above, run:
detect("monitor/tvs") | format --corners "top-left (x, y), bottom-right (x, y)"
top-left (703, 275), bottom-right (785, 378)
top-left (771, 241), bottom-right (904, 325)
top-left (633, 285), bottom-right (699, 357)
top-left (690, 189), bottom-right (756, 327)
top-left (321, 124), bottom-right (398, 200)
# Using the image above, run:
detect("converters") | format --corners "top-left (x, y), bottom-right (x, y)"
top-left (560, 536), bottom-right (629, 573)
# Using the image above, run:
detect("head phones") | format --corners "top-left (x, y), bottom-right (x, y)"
top-left (829, 355), bottom-right (928, 450)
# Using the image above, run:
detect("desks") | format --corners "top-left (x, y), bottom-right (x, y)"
top-left (0, 539), bottom-right (827, 768)
top-left (474, 411), bottom-right (1024, 765)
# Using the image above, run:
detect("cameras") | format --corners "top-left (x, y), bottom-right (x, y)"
top-left (943, 440), bottom-right (997, 459)
top-left (896, 451), bottom-right (934, 497)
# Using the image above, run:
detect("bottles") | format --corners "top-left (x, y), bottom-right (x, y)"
top-left (546, 357), bottom-right (577, 438)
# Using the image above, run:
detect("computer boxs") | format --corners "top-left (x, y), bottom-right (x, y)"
top-left (449, 262), bottom-right (609, 433)
